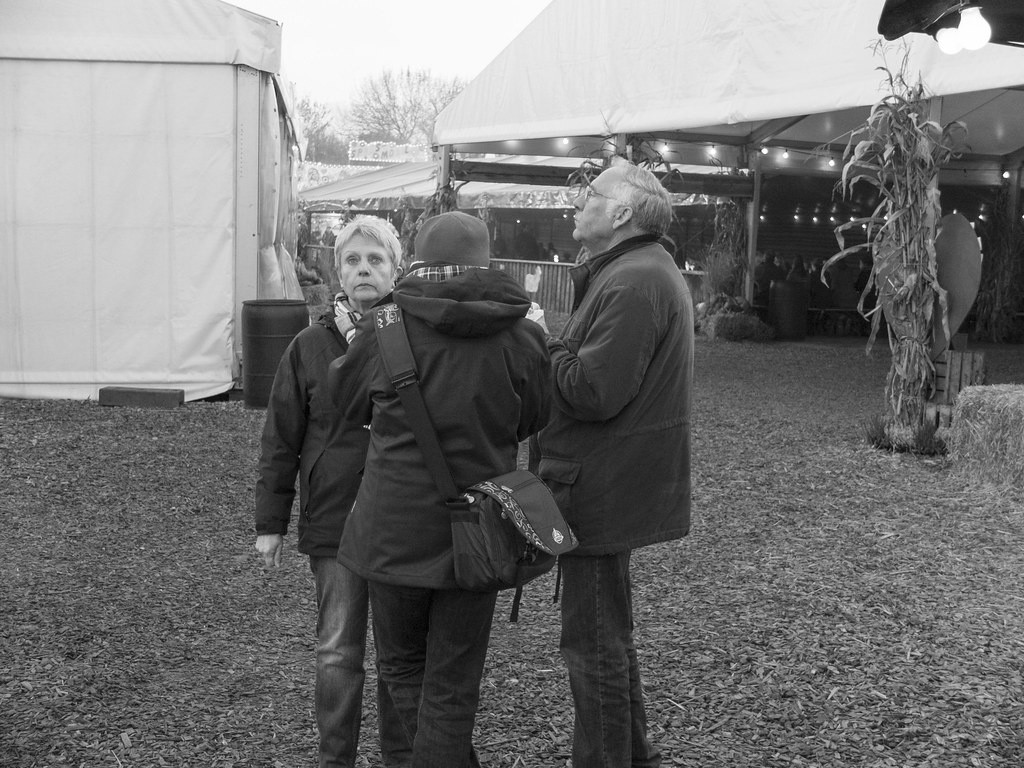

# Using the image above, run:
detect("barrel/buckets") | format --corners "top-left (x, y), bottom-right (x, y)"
top-left (240, 295), bottom-right (312, 412)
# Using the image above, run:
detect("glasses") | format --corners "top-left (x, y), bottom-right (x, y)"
top-left (583, 185), bottom-right (627, 208)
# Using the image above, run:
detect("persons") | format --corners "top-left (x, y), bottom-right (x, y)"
top-left (327, 211), bottom-right (550, 768)
top-left (540, 164), bottom-right (696, 768)
top-left (251, 215), bottom-right (424, 768)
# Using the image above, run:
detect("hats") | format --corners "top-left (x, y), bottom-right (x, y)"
top-left (413, 212), bottom-right (489, 268)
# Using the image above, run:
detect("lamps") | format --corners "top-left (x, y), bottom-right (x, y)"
top-left (955, 0), bottom-right (992, 51)
top-left (927, 23), bottom-right (961, 54)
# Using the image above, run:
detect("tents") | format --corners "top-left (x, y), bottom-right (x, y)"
top-left (429, 0), bottom-right (1024, 324)
top-left (296, 159), bottom-right (736, 290)
top-left (0, 1), bottom-right (289, 404)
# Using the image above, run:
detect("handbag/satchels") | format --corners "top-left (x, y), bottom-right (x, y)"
top-left (370, 303), bottom-right (581, 594)
